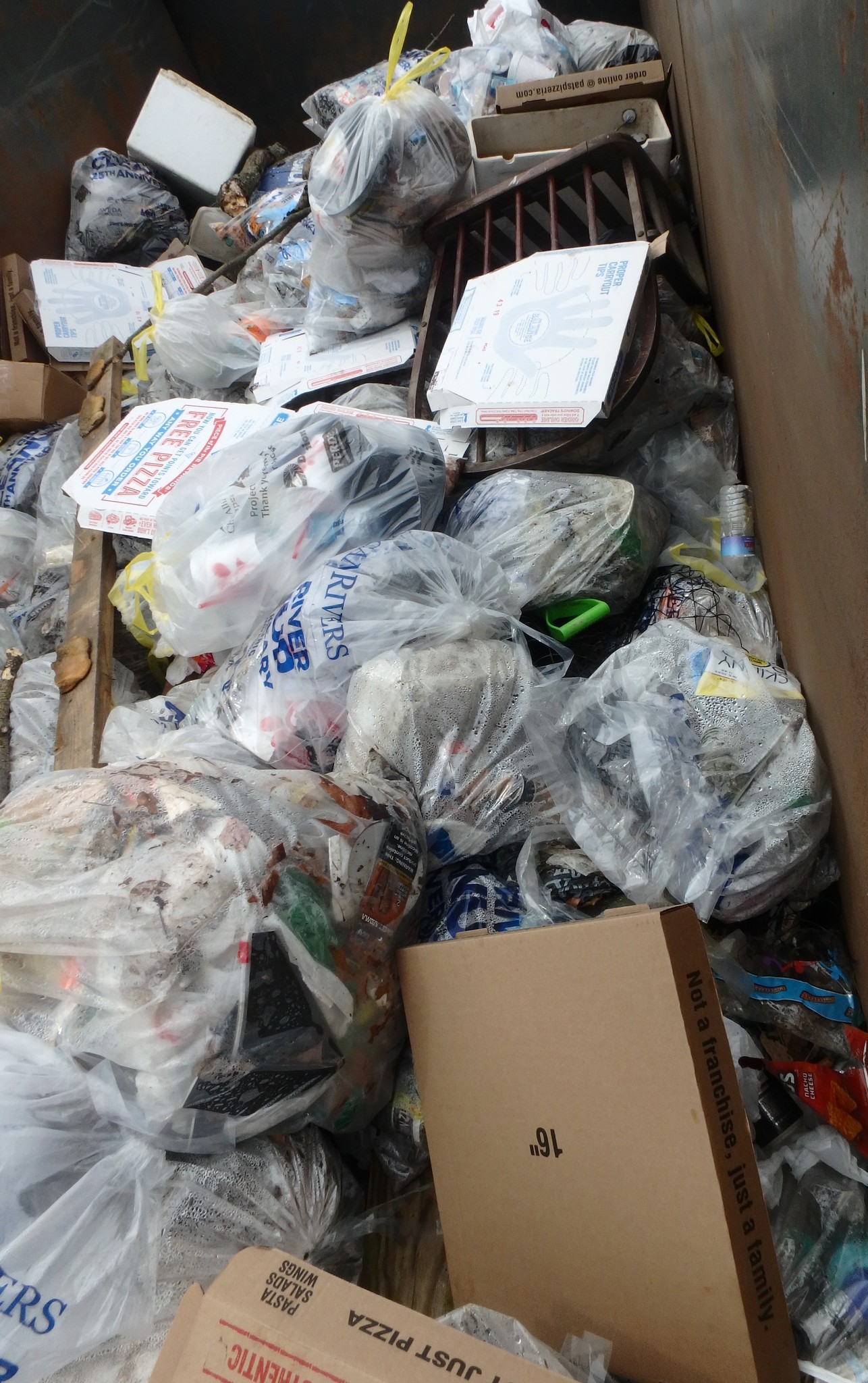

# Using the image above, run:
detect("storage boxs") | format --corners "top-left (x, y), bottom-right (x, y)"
top-left (144, 1243), bottom-right (576, 1383)
top-left (126, 68), bottom-right (257, 204)
top-left (0, 230), bottom-right (667, 540)
top-left (466, 61), bottom-right (674, 201)
top-left (397, 901), bottom-right (803, 1383)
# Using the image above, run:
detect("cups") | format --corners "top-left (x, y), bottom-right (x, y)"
top-left (751, 1068), bottom-right (806, 1151)
top-left (791, 1266), bottom-right (868, 1363)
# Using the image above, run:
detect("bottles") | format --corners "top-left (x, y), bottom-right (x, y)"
top-left (718, 483), bottom-right (757, 590)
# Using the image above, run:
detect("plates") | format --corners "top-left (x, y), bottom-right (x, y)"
top-left (307, 100), bottom-right (392, 218)
